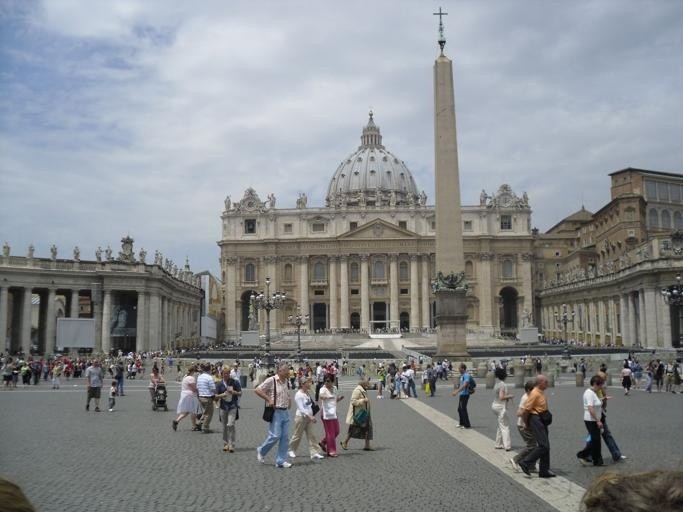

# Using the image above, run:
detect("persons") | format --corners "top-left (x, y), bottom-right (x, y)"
top-left (50, 243), bottom-right (58, 261)
top-left (387, 189), bottom-right (397, 207)
top-left (578, 358), bottom-right (586, 386)
top-left (95, 246), bottom-right (104, 261)
top-left (106, 380), bottom-right (118, 413)
top-left (376, 189), bottom-right (383, 206)
top-left (252, 360), bottom-right (293, 468)
top-left (84, 357), bottom-right (103, 414)
top-left (286, 375), bottom-right (326, 460)
top-left (491, 367), bottom-right (515, 452)
top-left (72, 245), bottom-right (80, 260)
top-left (286, 359), bottom-right (365, 389)
top-left (316, 372), bottom-right (345, 458)
top-left (339, 191), bottom-right (347, 208)
top-left (0, 347), bottom-right (165, 398)
top-left (105, 246), bottom-right (113, 260)
top-left (166, 340), bottom-right (235, 356)
top-left (314, 327), bottom-right (438, 334)
top-left (576, 468), bottom-right (683, 512)
top-left (299, 193), bottom-right (307, 209)
top-left (224, 194), bottom-right (230, 214)
top-left (478, 189), bottom-right (488, 205)
top-left (339, 379), bottom-right (374, 453)
top-left (490, 357), bottom-right (542, 375)
top-left (327, 190), bottom-right (336, 209)
top-left (139, 247), bottom-right (147, 263)
top-left (575, 375), bottom-right (609, 468)
top-left (522, 373), bottom-right (557, 479)
top-left (585, 371), bottom-right (628, 464)
top-left (376, 358), bottom-right (450, 399)
top-left (508, 379), bottom-right (540, 474)
top-left (267, 193), bottom-right (276, 209)
top-left (522, 189), bottom-right (529, 204)
top-left (153, 250), bottom-right (202, 288)
top-left (450, 363), bottom-right (473, 431)
top-left (0, 476), bottom-right (36, 512)
top-left (3, 240), bottom-right (10, 255)
top-left (405, 190), bottom-right (415, 206)
top-left (418, 189), bottom-right (426, 205)
top-left (541, 335), bottom-right (588, 347)
top-left (622, 358), bottom-right (682, 396)
top-left (169, 362), bottom-right (242, 454)
top-left (26, 242), bottom-right (36, 259)
top-left (229, 355), bottom-right (284, 386)
top-left (356, 190), bottom-right (367, 207)
top-left (146, 367), bottom-right (166, 410)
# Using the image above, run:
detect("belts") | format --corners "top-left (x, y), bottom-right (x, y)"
top-left (278, 408), bottom-right (287, 410)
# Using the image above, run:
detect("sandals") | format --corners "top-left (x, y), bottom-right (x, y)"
top-left (310, 440), bottom-right (338, 459)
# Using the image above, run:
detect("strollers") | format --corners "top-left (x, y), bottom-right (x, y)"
top-left (151, 380), bottom-right (169, 412)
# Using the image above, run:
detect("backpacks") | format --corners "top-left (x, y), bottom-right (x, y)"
top-left (463, 373), bottom-right (476, 394)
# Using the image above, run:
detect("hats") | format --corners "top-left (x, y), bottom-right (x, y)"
top-left (298, 375), bottom-right (312, 384)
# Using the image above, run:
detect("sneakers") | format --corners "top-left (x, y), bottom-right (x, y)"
top-left (276, 450), bottom-right (296, 468)
top-left (578, 455), bottom-right (627, 466)
top-left (223, 442), bottom-right (234, 452)
top-left (340, 441), bottom-right (347, 450)
top-left (257, 447), bottom-right (265, 464)
top-left (363, 446), bottom-right (375, 451)
top-left (510, 457), bottom-right (556, 477)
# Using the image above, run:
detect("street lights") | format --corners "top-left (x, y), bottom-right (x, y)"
top-left (659, 271), bottom-right (683, 378)
top-left (285, 312), bottom-right (310, 363)
top-left (249, 275), bottom-right (287, 369)
top-left (553, 303), bottom-right (576, 359)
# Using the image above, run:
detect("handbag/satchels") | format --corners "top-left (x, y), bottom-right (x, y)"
top-left (539, 410), bottom-right (552, 426)
top-left (353, 408), bottom-right (369, 425)
top-left (310, 397), bottom-right (319, 415)
top-left (322, 394), bottom-right (337, 420)
top-left (263, 407), bottom-right (275, 423)
